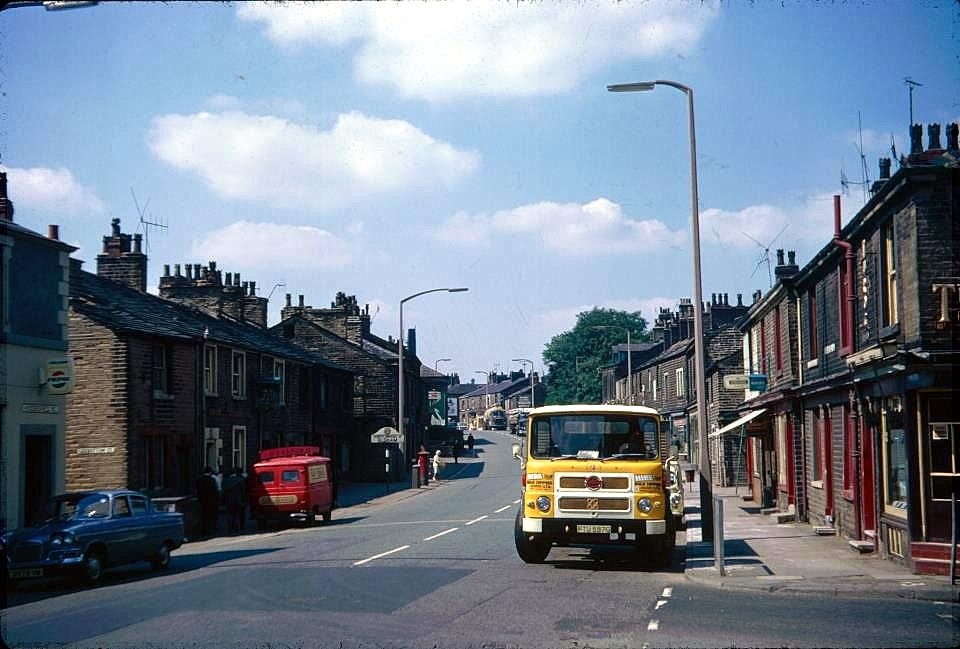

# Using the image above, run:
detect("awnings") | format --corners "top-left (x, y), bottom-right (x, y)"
top-left (692, 408), bottom-right (769, 444)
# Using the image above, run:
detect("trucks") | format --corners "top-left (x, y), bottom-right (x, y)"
top-left (482, 407), bottom-right (507, 431)
top-left (508, 407), bottom-right (535, 437)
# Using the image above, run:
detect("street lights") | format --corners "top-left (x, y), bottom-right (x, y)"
top-left (511, 358), bottom-right (535, 408)
top-left (434, 358), bottom-right (453, 376)
top-left (591, 325), bottom-right (632, 405)
top-left (606, 79), bottom-right (715, 544)
top-left (395, 287), bottom-right (469, 482)
top-left (475, 370), bottom-right (488, 409)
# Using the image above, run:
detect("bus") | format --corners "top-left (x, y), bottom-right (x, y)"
top-left (511, 403), bottom-right (685, 565)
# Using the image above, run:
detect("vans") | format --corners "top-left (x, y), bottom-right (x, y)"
top-left (246, 445), bottom-right (332, 530)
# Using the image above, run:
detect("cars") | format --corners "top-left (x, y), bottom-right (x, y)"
top-left (0, 489), bottom-right (186, 593)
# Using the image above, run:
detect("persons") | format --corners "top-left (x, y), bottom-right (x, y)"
top-left (468, 436), bottom-right (473, 450)
top-left (452, 439), bottom-right (460, 464)
top-left (198, 465), bottom-right (250, 540)
top-left (670, 434), bottom-right (681, 450)
top-left (431, 449), bottom-right (442, 482)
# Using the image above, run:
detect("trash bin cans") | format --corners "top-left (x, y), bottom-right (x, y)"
top-left (411, 464), bottom-right (420, 488)
top-left (685, 470), bottom-right (695, 482)
